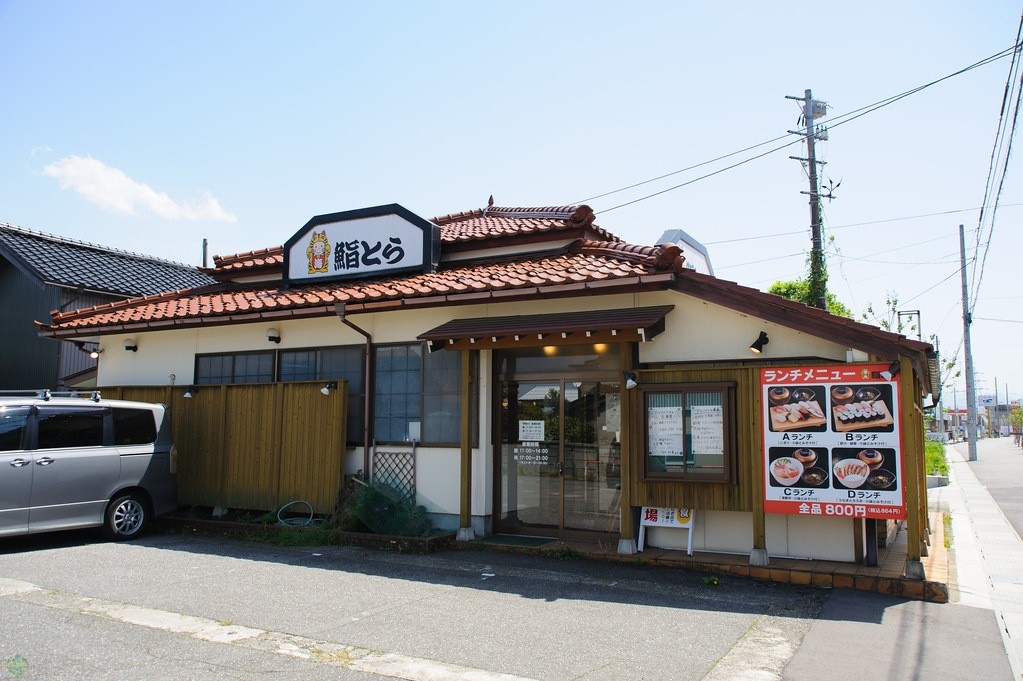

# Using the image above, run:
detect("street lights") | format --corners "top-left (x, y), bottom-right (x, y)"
top-left (950, 388), bottom-right (957, 444)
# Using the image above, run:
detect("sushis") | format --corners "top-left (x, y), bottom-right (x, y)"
top-left (836, 407), bottom-right (886, 424)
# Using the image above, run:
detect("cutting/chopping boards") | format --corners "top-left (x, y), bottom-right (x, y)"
top-left (832, 399), bottom-right (894, 431)
top-left (770, 400), bottom-right (827, 432)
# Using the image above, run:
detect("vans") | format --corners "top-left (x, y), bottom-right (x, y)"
top-left (0, 390), bottom-right (187, 542)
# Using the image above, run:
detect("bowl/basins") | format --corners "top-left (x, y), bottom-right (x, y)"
top-left (856, 449), bottom-right (885, 470)
top-left (791, 448), bottom-right (818, 468)
top-left (769, 387), bottom-right (791, 405)
top-left (833, 458), bottom-right (871, 489)
top-left (792, 387), bottom-right (816, 402)
top-left (856, 386), bottom-right (882, 402)
top-left (770, 457), bottom-right (804, 486)
top-left (800, 466), bottom-right (828, 486)
top-left (832, 386), bottom-right (855, 405)
top-left (866, 468), bottom-right (896, 489)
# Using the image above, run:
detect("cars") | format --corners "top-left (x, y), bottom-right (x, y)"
top-left (606, 437), bottom-right (622, 489)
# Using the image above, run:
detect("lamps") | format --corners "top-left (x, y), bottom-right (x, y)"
top-left (90, 348), bottom-right (104, 358)
top-left (880, 362), bottom-right (900, 380)
top-left (123, 338), bottom-right (138, 353)
top-left (622, 370), bottom-right (637, 389)
top-left (749, 330), bottom-right (770, 354)
top-left (266, 327), bottom-right (281, 344)
